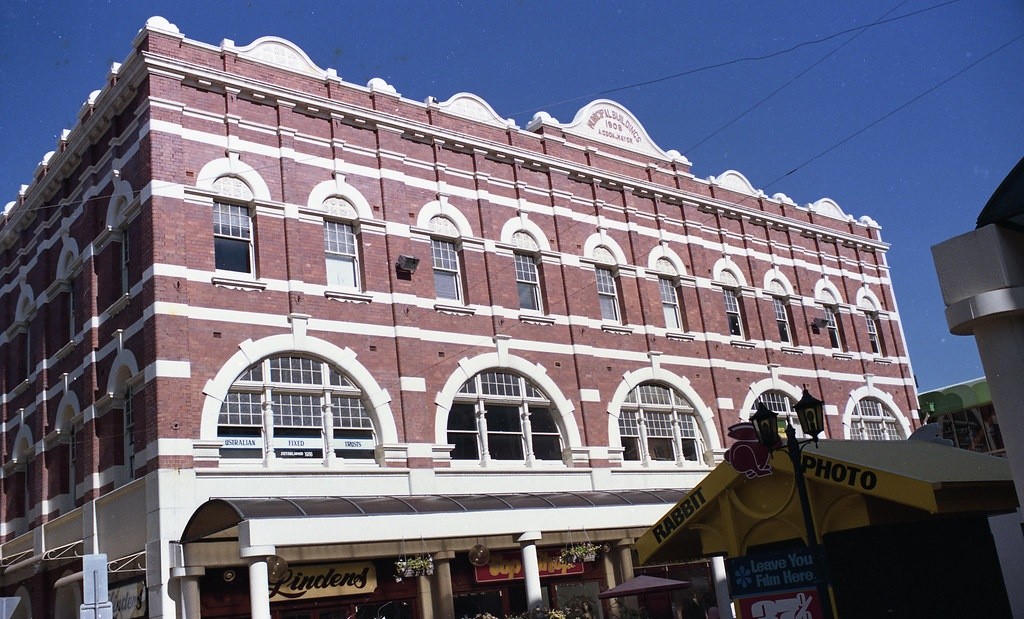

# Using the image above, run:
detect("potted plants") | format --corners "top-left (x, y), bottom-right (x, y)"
top-left (578, 542), bottom-right (600, 562)
top-left (396, 559), bottom-right (415, 577)
top-left (412, 554), bottom-right (434, 576)
top-left (562, 547), bottom-right (581, 563)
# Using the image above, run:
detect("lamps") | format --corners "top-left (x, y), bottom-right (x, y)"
top-left (394, 254), bottom-right (421, 275)
top-left (599, 542), bottom-right (611, 553)
top-left (810, 317), bottom-right (829, 328)
top-left (223, 569), bottom-right (236, 581)
